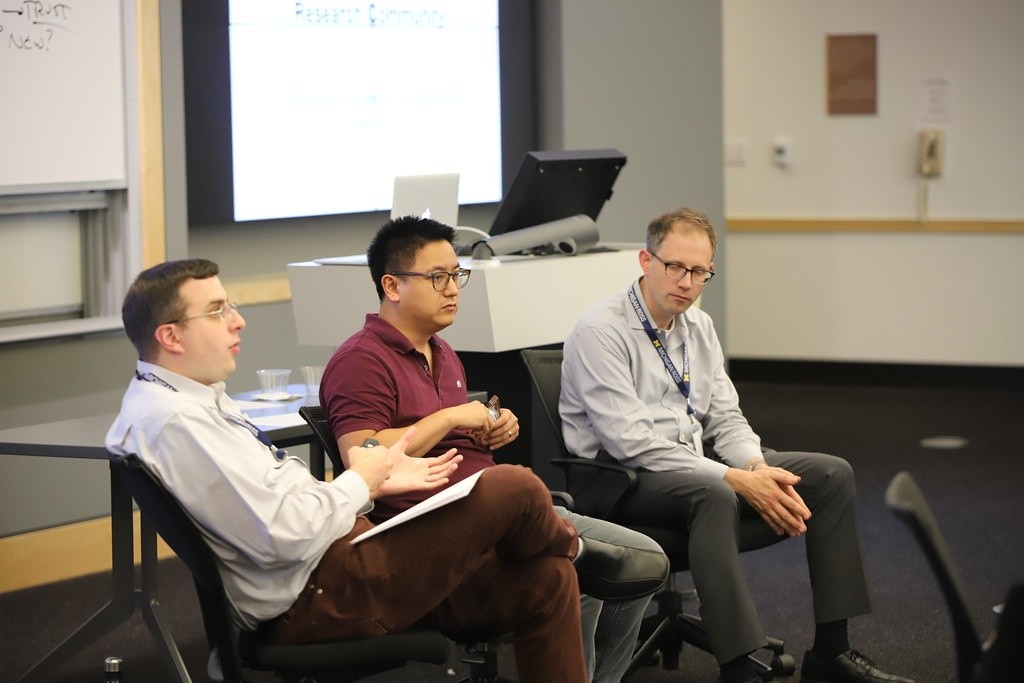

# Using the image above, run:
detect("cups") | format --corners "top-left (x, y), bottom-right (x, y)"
top-left (256, 369), bottom-right (291, 396)
top-left (300, 365), bottom-right (325, 397)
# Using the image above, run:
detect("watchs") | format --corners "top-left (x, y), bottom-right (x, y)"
top-left (749, 460), bottom-right (767, 472)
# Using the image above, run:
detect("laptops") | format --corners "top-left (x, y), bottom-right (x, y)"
top-left (312, 173), bottom-right (460, 266)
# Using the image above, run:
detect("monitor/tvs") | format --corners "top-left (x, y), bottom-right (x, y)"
top-left (490, 147), bottom-right (627, 237)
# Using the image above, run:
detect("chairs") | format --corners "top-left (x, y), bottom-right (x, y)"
top-left (520, 348), bottom-right (798, 683)
top-left (885, 472), bottom-right (924, 550)
top-left (299, 406), bottom-right (576, 683)
top-left (109, 451), bottom-right (450, 683)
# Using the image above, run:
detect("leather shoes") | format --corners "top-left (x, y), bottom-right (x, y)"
top-left (800, 650), bottom-right (916, 683)
top-left (573, 536), bottom-right (670, 604)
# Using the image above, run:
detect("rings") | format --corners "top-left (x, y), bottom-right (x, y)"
top-left (508, 431), bottom-right (512, 438)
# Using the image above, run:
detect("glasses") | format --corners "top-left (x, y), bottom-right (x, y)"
top-left (163, 303), bottom-right (238, 323)
top-left (391, 268), bottom-right (471, 292)
top-left (648, 250), bottom-right (715, 285)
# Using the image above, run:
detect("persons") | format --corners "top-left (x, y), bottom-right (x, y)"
top-left (106, 258), bottom-right (669, 683)
top-left (320, 216), bottom-right (671, 683)
top-left (559, 208), bottom-right (919, 683)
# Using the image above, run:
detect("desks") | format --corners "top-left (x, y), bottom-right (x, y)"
top-left (0, 382), bottom-right (486, 683)
